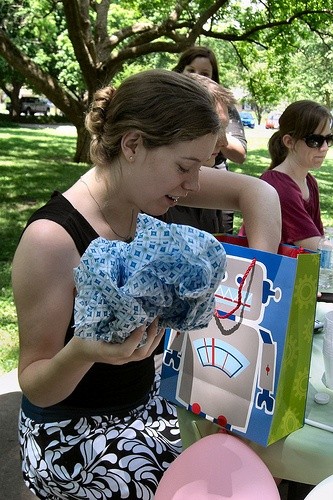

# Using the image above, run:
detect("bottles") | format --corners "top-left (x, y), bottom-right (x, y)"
top-left (317, 227), bottom-right (333, 292)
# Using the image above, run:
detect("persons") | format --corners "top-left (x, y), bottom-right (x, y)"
top-left (140, 73), bottom-right (235, 234)
top-left (239, 99), bottom-right (332, 252)
top-left (11, 69), bottom-right (281, 500)
top-left (171, 48), bottom-right (247, 234)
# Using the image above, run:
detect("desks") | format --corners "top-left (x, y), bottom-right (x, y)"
top-left (175, 297), bottom-right (332, 486)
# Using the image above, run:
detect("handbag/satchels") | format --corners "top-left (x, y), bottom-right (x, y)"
top-left (160, 234), bottom-right (323, 447)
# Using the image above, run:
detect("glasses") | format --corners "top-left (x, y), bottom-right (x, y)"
top-left (291, 133), bottom-right (333, 148)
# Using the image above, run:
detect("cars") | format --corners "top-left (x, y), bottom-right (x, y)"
top-left (238, 112), bottom-right (255, 129)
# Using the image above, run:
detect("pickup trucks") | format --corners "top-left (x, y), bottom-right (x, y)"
top-left (6, 96), bottom-right (51, 117)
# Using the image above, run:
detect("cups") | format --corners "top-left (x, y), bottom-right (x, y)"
top-left (322, 310), bottom-right (333, 390)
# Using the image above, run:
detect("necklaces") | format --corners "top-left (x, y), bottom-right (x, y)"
top-left (80, 176), bottom-right (134, 244)
